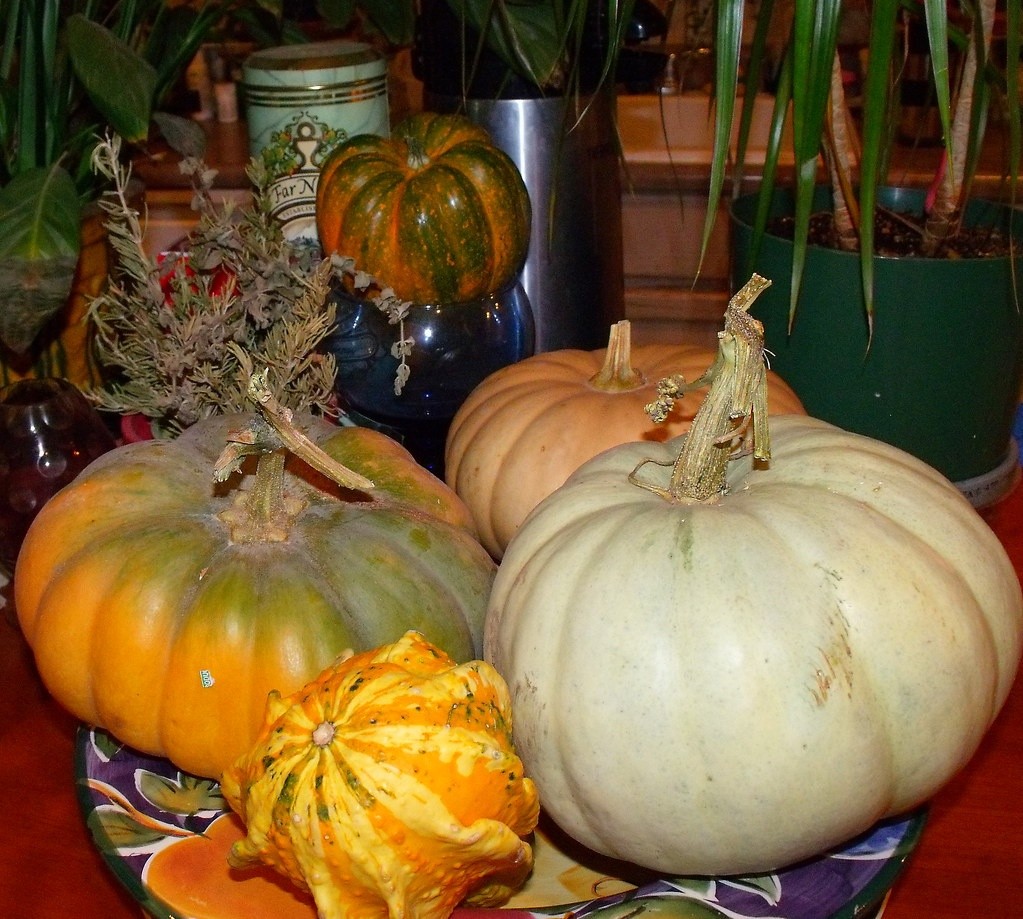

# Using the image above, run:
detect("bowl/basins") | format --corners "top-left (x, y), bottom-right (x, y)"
top-left (325, 289), bottom-right (535, 428)
top-left (0, 379), bottom-right (116, 567)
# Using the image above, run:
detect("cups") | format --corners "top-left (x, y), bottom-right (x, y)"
top-left (242, 40), bottom-right (390, 257)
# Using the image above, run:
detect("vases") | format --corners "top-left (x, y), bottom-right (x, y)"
top-left (244, 49), bottom-right (390, 214)
top-left (1, 375), bottom-right (118, 577)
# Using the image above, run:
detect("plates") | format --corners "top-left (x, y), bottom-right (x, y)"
top-left (77, 724), bottom-right (924, 919)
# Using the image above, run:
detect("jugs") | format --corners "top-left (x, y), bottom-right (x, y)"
top-left (411, 0), bottom-right (665, 353)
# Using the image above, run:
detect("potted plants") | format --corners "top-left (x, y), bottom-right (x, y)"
top-left (429, 0), bottom-right (625, 367)
top-left (680, 0), bottom-right (1023, 507)
top-left (0, 1), bottom-right (236, 400)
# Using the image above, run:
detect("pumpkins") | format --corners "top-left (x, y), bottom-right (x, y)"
top-left (227, 631), bottom-right (542, 919)
top-left (482, 273), bottom-right (1023, 877)
top-left (442, 318), bottom-right (807, 563)
top-left (13, 374), bottom-right (501, 781)
top-left (314, 110), bottom-right (532, 306)
